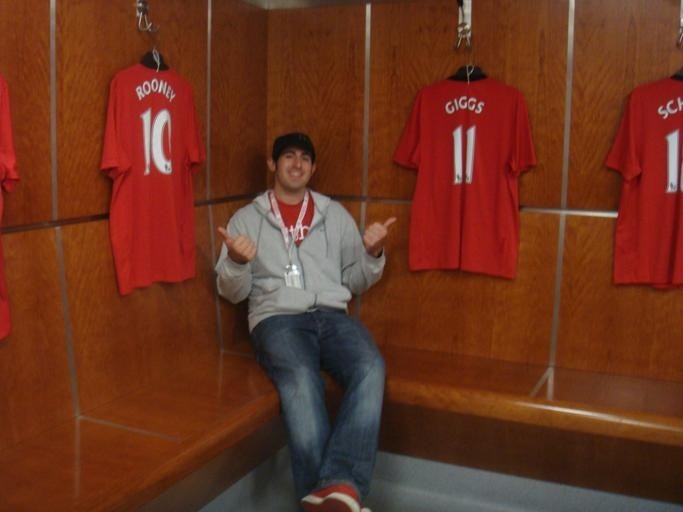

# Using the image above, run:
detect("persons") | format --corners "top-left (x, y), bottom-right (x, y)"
top-left (212, 130), bottom-right (397, 511)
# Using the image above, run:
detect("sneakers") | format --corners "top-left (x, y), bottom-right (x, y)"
top-left (300, 484), bottom-right (361, 512)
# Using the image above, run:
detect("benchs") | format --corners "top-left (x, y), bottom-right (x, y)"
top-left (0, 343), bottom-right (683, 512)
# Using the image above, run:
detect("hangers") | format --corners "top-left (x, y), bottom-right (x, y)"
top-left (463, 45), bottom-right (475, 66)
top-left (149, 25), bottom-right (162, 50)
